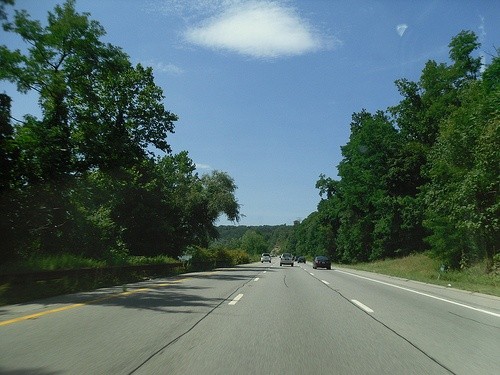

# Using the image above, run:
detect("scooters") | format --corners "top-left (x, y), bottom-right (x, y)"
top-left (276, 254), bottom-right (277, 257)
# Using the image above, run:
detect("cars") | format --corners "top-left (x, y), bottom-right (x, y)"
top-left (270, 254), bottom-right (275, 257)
top-left (314, 256), bottom-right (331, 270)
top-left (292, 255), bottom-right (297, 261)
top-left (279, 255), bottom-right (282, 258)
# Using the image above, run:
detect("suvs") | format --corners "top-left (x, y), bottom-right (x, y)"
top-left (261, 253), bottom-right (271, 263)
top-left (280, 253), bottom-right (294, 267)
top-left (298, 256), bottom-right (306, 263)
